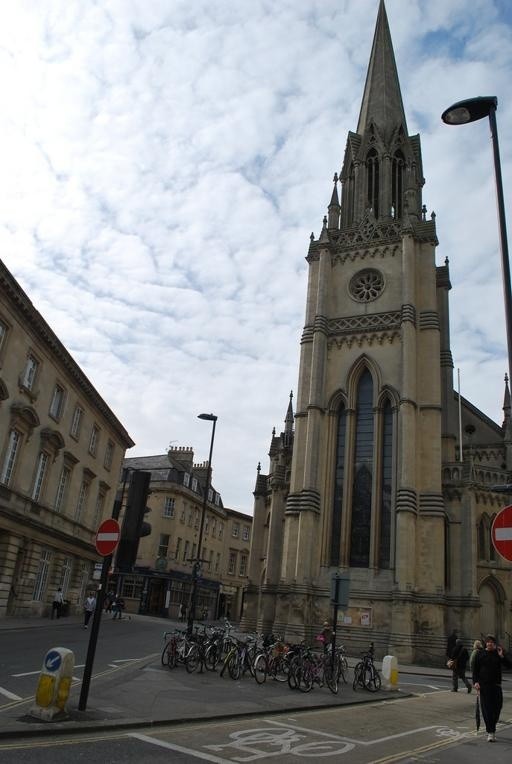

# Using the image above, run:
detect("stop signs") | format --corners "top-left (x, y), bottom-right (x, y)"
top-left (94, 518), bottom-right (122, 556)
top-left (491, 503), bottom-right (512, 562)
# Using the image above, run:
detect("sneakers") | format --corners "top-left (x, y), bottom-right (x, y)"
top-left (450, 688), bottom-right (457, 692)
top-left (468, 686), bottom-right (472, 693)
top-left (488, 732), bottom-right (497, 742)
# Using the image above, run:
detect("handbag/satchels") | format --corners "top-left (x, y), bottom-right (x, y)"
top-left (446, 659), bottom-right (457, 670)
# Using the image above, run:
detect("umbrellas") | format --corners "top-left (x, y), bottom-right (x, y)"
top-left (475, 688), bottom-right (481, 734)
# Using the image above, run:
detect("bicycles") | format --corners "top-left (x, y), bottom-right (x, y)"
top-left (161, 617), bottom-right (382, 694)
top-left (109, 602), bottom-right (132, 625)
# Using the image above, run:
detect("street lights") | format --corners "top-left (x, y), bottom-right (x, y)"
top-left (182, 413), bottom-right (218, 659)
top-left (441, 95), bottom-right (512, 400)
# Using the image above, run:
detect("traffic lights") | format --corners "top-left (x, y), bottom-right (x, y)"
top-left (115, 471), bottom-right (153, 569)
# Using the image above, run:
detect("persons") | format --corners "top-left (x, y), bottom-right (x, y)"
top-left (83, 592), bottom-right (96, 627)
top-left (112, 595), bottom-right (125, 620)
top-left (320, 621), bottom-right (331, 652)
top-left (177, 600), bottom-right (184, 621)
top-left (450, 639), bottom-right (471, 693)
top-left (473, 635), bottom-right (510, 742)
top-left (51, 588), bottom-right (64, 619)
top-left (470, 640), bottom-right (483, 671)
top-left (106, 590), bottom-right (115, 612)
top-left (181, 603), bottom-right (188, 621)
